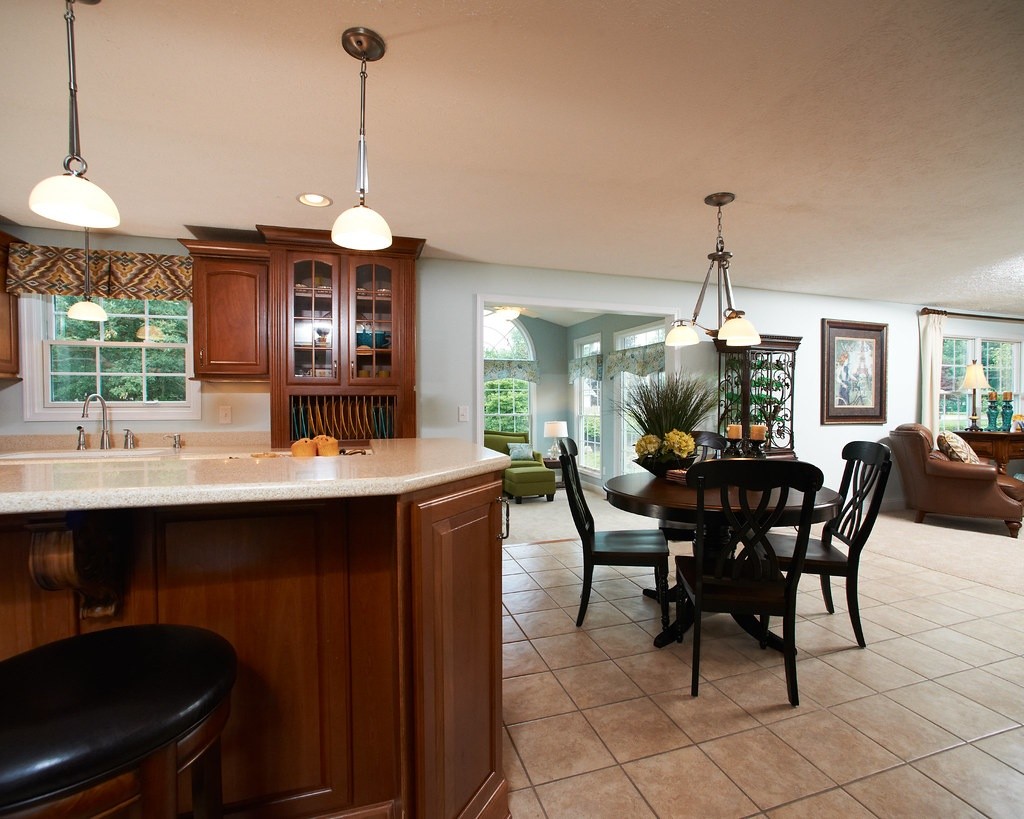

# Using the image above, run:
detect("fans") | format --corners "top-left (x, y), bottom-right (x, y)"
top-left (483, 305), bottom-right (540, 321)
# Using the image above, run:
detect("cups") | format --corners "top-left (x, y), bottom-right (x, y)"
top-left (317, 286), bottom-right (332, 293)
top-left (357, 288), bottom-right (369, 296)
top-left (291, 435), bottom-right (339, 457)
top-left (378, 288), bottom-right (392, 296)
top-left (295, 284), bottom-right (308, 292)
top-left (359, 370), bottom-right (370, 378)
top-left (379, 371), bottom-right (390, 377)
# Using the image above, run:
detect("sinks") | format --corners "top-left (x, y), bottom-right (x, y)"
top-left (0, 451), bottom-right (169, 457)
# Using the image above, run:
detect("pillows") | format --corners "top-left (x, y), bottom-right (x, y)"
top-left (507, 442), bottom-right (534, 460)
top-left (937, 429), bottom-right (980, 464)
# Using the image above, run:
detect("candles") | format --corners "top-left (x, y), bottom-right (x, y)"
top-left (750, 424), bottom-right (768, 439)
top-left (727, 424), bottom-right (743, 438)
top-left (1002, 391), bottom-right (1013, 400)
top-left (988, 390), bottom-right (997, 400)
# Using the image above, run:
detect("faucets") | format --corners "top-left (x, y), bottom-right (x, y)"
top-left (82, 393), bottom-right (109, 450)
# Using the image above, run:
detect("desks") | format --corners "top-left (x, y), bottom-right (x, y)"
top-left (604, 471), bottom-right (843, 656)
top-left (953, 429), bottom-right (1024, 475)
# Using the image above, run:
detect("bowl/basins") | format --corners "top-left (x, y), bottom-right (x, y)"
top-left (356, 331), bottom-right (391, 348)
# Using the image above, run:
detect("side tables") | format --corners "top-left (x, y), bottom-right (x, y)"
top-left (543, 457), bottom-right (567, 488)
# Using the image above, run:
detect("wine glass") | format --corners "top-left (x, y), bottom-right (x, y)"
top-left (316, 327), bottom-right (330, 347)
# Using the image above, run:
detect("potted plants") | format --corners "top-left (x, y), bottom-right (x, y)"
top-left (606, 366), bottom-right (727, 475)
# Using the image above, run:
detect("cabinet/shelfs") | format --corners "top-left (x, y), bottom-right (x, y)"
top-left (0, 232), bottom-right (29, 386)
top-left (180, 227), bottom-right (432, 437)
top-left (410, 481), bottom-right (512, 819)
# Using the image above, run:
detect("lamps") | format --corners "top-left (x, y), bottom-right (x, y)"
top-left (28, 0), bottom-right (120, 229)
top-left (331, 26), bottom-right (393, 250)
top-left (67, 227), bottom-right (109, 322)
top-left (955, 359), bottom-right (991, 431)
top-left (544, 420), bottom-right (568, 460)
top-left (665, 190), bottom-right (762, 346)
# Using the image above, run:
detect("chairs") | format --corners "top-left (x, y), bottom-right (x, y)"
top-left (743, 442), bottom-right (892, 648)
top-left (560, 431), bottom-right (726, 630)
top-left (682, 461), bottom-right (825, 705)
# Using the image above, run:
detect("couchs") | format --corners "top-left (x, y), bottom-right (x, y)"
top-left (484, 429), bottom-right (545, 490)
top-left (889, 422), bottom-right (1024, 538)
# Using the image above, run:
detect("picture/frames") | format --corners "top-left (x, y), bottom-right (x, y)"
top-left (820, 318), bottom-right (888, 424)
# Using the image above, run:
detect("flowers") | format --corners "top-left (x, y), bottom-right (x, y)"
top-left (634, 429), bottom-right (695, 469)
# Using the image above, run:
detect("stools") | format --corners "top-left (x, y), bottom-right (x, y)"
top-left (505, 467), bottom-right (556, 504)
top-left (0, 599), bottom-right (240, 819)
